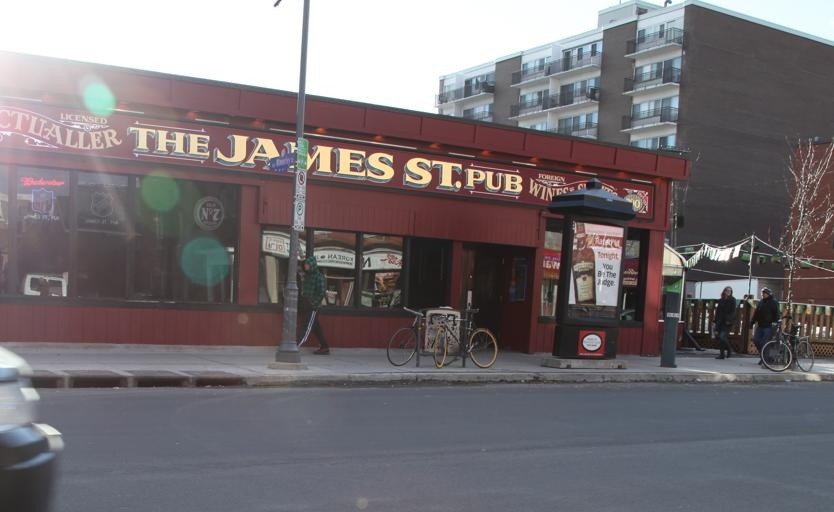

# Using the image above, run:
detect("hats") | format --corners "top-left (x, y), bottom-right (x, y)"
top-left (761, 287), bottom-right (772, 297)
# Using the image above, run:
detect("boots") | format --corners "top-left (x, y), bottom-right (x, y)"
top-left (716, 347), bottom-right (724, 359)
top-left (727, 346), bottom-right (731, 358)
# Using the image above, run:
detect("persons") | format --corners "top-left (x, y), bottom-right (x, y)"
top-left (715, 286), bottom-right (737, 359)
top-left (750, 287), bottom-right (780, 365)
top-left (298, 257), bottom-right (330, 354)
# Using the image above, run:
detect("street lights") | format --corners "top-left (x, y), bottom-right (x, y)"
top-left (271, 0), bottom-right (312, 366)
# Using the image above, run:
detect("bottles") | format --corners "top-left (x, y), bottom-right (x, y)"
top-left (542, 275), bottom-right (555, 317)
top-left (571, 221), bottom-right (596, 304)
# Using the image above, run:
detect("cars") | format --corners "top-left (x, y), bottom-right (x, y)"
top-left (0, 341), bottom-right (68, 512)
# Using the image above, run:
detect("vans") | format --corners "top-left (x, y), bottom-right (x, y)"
top-left (19, 271), bottom-right (70, 296)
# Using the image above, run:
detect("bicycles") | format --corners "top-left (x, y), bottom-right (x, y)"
top-left (429, 304), bottom-right (500, 371)
top-left (386, 304), bottom-right (463, 366)
top-left (759, 316), bottom-right (817, 372)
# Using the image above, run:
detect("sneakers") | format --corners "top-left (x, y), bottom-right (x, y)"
top-left (314, 347), bottom-right (328, 355)
